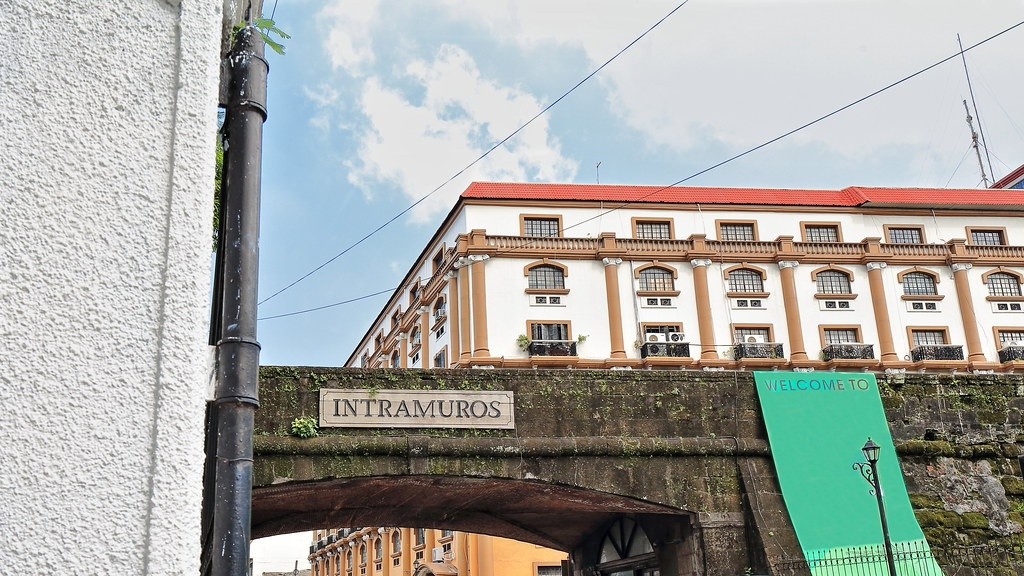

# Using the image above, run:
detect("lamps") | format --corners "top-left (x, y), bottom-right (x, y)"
top-left (413, 559), bottom-right (419, 571)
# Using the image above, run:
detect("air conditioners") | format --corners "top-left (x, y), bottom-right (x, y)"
top-left (668, 332), bottom-right (685, 341)
top-left (743, 335), bottom-right (765, 358)
top-left (841, 345), bottom-right (861, 357)
top-left (433, 547), bottom-right (444, 561)
top-left (435, 309), bottom-right (445, 319)
top-left (1003, 341), bottom-right (1024, 349)
top-left (412, 338), bottom-right (420, 347)
top-left (645, 332), bottom-right (665, 357)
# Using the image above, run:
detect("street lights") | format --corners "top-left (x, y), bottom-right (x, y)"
top-left (852, 437), bottom-right (897, 576)
